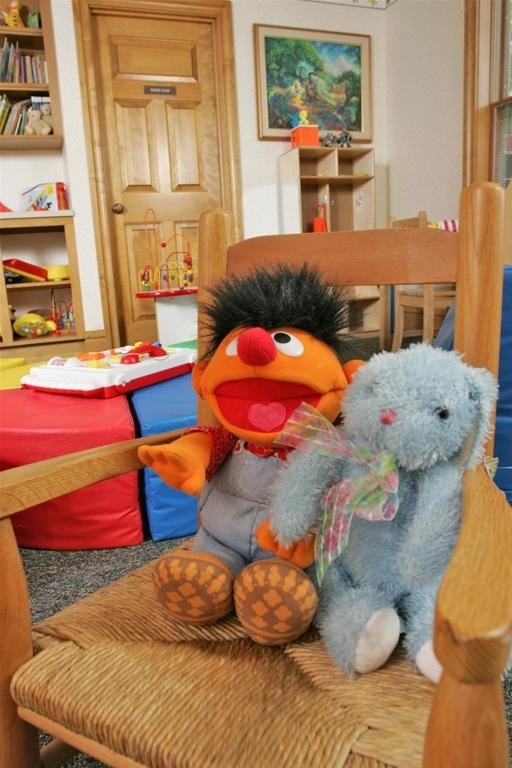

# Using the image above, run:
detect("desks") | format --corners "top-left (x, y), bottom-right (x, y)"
top-left (135, 285), bottom-right (198, 347)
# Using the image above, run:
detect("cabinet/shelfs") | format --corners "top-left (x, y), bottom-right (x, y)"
top-left (0, 0), bottom-right (65, 150)
top-left (0, 209), bottom-right (86, 347)
top-left (278, 147), bottom-right (382, 337)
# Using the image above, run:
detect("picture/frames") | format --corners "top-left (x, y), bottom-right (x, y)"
top-left (253, 22), bottom-right (373, 143)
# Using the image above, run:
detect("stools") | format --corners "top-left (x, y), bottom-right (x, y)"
top-left (163, 338), bottom-right (198, 352)
top-left (0, 356), bottom-right (26, 370)
top-left (0, 387), bottom-right (145, 551)
top-left (130, 373), bottom-right (204, 541)
top-left (0, 360), bottom-right (48, 389)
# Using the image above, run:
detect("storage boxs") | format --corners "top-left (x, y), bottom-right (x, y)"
top-left (288, 124), bottom-right (320, 148)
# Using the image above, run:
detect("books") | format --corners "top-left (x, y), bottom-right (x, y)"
top-left (0, 34), bottom-right (55, 139)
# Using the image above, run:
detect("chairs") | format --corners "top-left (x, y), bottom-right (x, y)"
top-left (429, 263), bottom-right (512, 504)
top-left (388, 210), bottom-right (456, 351)
top-left (0, 179), bottom-right (512, 768)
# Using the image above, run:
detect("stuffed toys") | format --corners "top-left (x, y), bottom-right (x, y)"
top-left (135, 259), bottom-right (367, 646)
top-left (320, 132), bottom-right (337, 147)
top-left (270, 342), bottom-right (512, 684)
top-left (337, 129), bottom-right (353, 147)
